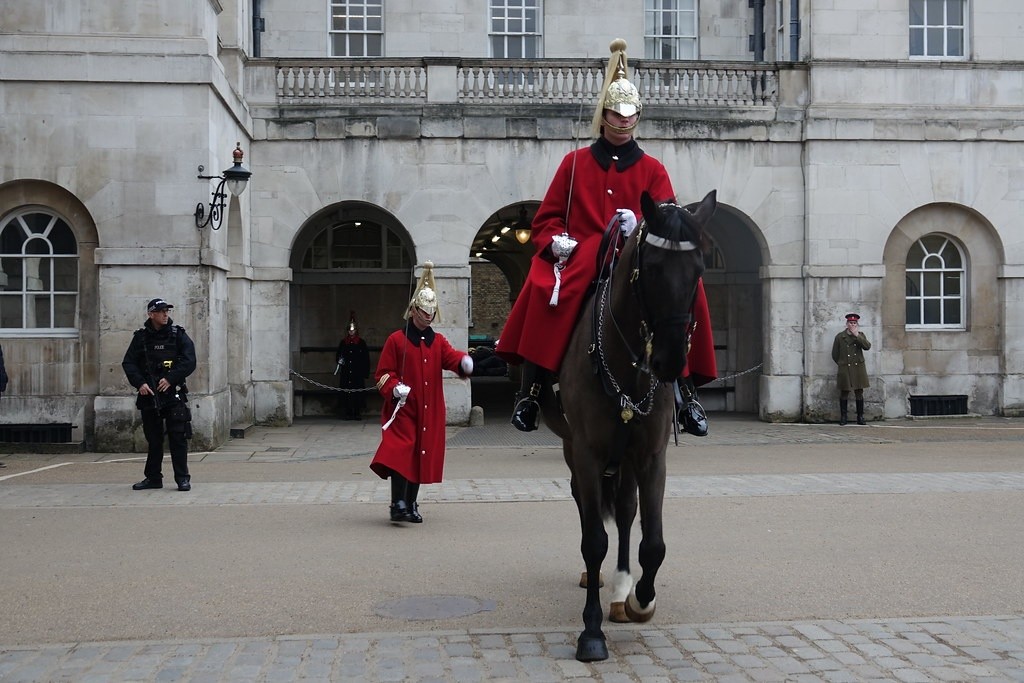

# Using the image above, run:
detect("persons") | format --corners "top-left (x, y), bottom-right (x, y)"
top-left (495, 80), bottom-right (718, 438)
top-left (369, 288), bottom-right (474, 524)
top-left (336, 323), bottom-right (372, 421)
top-left (0, 344), bottom-right (9, 469)
top-left (832, 314), bottom-right (872, 425)
top-left (121, 298), bottom-right (197, 490)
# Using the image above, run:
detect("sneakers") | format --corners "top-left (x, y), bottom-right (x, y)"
top-left (178, 479), bottom-right (190, 490)
top-left (133, 478), bottom-right (163, 490)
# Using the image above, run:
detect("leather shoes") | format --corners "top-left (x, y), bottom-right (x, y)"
top-left (513, 403), bottom-right (536, 432)
top-left (390, 500), bottom-right (412, 521)
top-left (410, 502), bottom-right (422, 523)
top-left (679, 407), bottom-right (708, 436)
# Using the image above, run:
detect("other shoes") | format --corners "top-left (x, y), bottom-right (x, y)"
top-left (354, 414), bottom-right (361, 421)
top-left (342, 416), bottom-right (348, 421)
top-left (0, 462), bottom-right (7, 467)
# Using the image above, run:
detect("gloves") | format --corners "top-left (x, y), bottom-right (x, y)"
top-left (461, 356), bottom-right (474, 373)
top-left (552, 241), bottom-right (562, 256)
top-left (617, 208), bottom-right (638, 236)
top-left (393, 387), bottom-right (402, 398)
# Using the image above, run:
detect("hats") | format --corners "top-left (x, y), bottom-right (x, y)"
top-left (845, 314), bottom-right (860, 322)
top-left (147, 299), bottom-right (173, 312)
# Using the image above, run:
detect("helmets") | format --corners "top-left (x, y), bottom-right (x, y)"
top-left (590, 38), bottom-right (642, 139)
top-left (346, 312), bottom-right (359, 331)
top-left (403, 260), bottom-right (441, 323)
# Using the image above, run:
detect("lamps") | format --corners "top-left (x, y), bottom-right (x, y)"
top-left (511, 203), bottom-right (532, 244)
top-left (194, 140), bottom-right (254, 232)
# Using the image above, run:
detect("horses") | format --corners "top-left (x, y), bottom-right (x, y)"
top-left (558, 187), bottom-right (718, 663)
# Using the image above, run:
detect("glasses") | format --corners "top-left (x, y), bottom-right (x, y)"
top-left (157, 310), bottom-right (171, 314)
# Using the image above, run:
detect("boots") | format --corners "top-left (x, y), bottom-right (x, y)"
top-left (840, 399), bottom-right (847, 424)
top-left (856, 399), bottom-right (866, 424)
